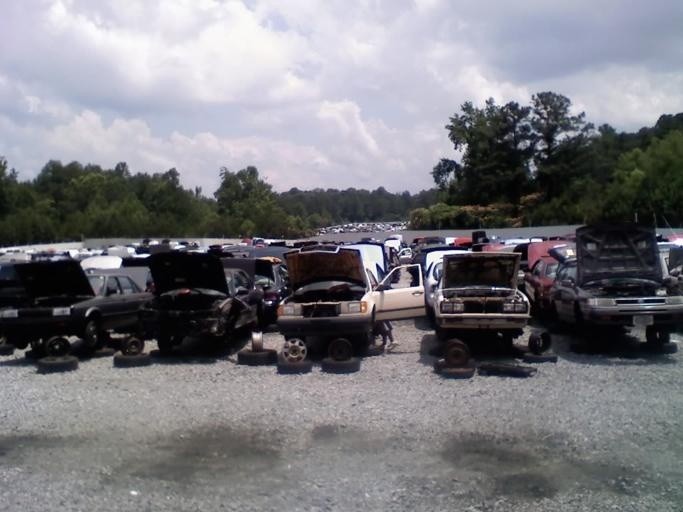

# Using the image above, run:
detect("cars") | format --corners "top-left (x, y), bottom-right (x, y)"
top-left (1, 246), bottom-right (154, 357)
